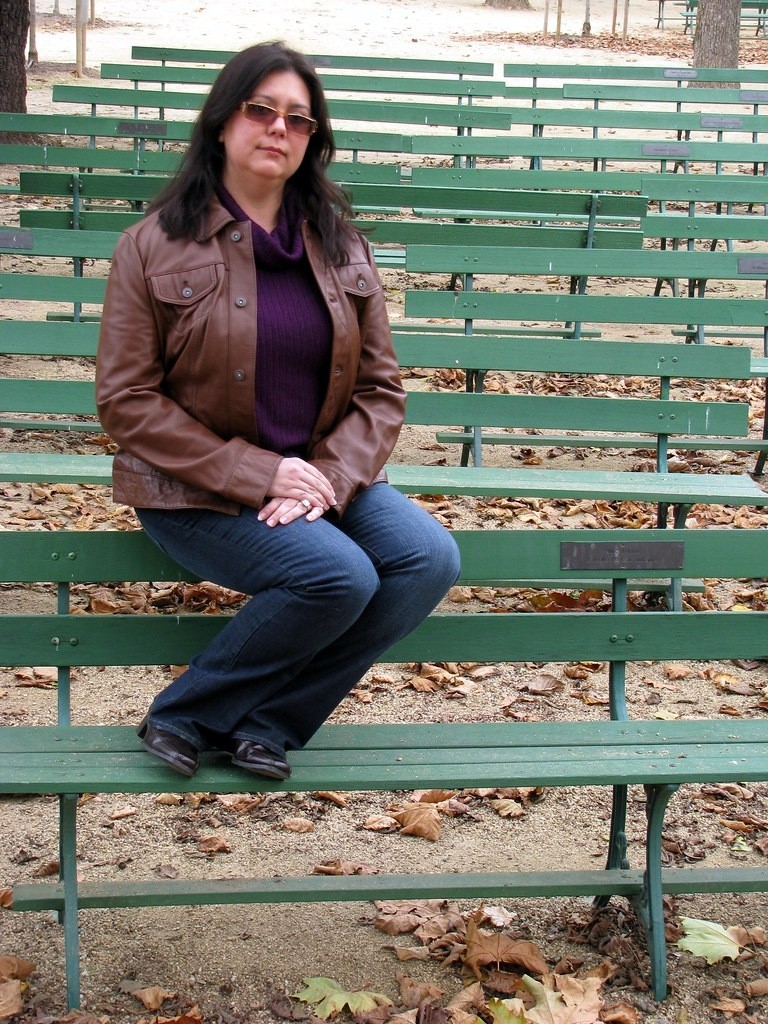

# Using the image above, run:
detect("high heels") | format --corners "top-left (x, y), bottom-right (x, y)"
top-left (134, 700), bottom-right (200, 778)
top-left (203, 730), bottom-right (292, 779)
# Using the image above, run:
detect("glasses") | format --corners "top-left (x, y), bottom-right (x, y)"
top-left (237, 101), bottom-right (319, 137)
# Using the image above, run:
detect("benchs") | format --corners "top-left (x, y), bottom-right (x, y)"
top-left (647, 0), bottom-right (768, 39)
top-left (0, 45), bottom-right (768, 1015)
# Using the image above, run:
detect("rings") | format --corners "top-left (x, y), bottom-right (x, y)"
top-left (301, 500), bottom-right (311, 511)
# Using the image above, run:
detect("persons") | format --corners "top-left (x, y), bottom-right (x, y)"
top-left (95, 41), bottom-right (460, 779)
